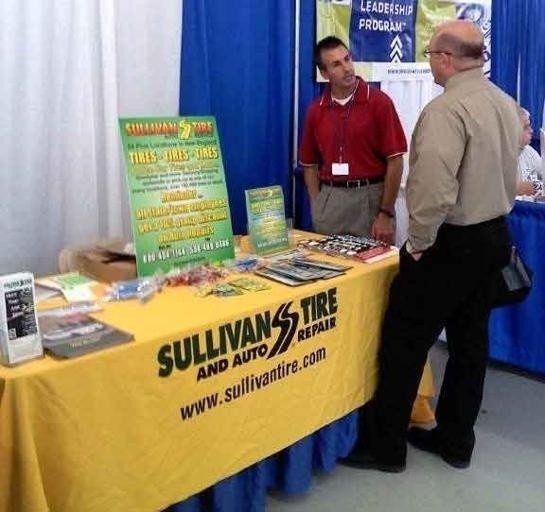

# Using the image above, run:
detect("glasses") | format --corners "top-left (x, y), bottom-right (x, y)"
top-left (423, 45), bottom-right (452, 58)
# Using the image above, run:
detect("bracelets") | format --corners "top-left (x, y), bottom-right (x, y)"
top-left (379, 208), bottom-right (394, 219)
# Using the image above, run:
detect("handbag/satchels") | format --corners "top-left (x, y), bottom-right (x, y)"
top-left (493, 246), bottom-right (533, 309)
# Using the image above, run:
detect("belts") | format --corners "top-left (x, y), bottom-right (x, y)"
top-left (320, 175), bottom-right (384, 190)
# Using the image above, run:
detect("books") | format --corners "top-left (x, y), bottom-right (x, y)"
top-left (302, 231), bottom-right (395, 265)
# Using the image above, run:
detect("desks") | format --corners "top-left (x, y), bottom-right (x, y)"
top-left (487, 199), bottom-right (545, 376)
top-left (0, 228), bottom-right (436, 512)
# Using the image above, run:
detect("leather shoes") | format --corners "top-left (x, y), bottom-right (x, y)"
top-left (337, 440), bottom-right (407, 472)
top-left (406, 425), bottom-right (475, 468)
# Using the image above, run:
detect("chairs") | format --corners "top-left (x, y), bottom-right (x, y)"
top-left (57, 241), bottom-right (96, 273)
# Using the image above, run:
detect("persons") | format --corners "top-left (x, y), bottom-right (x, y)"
top-left (336, 21), bottom-right (521, 475)
top-left (297, 36), bottom-right (408, 246)
top-left (514, 106), bottom-right (544, 203)
